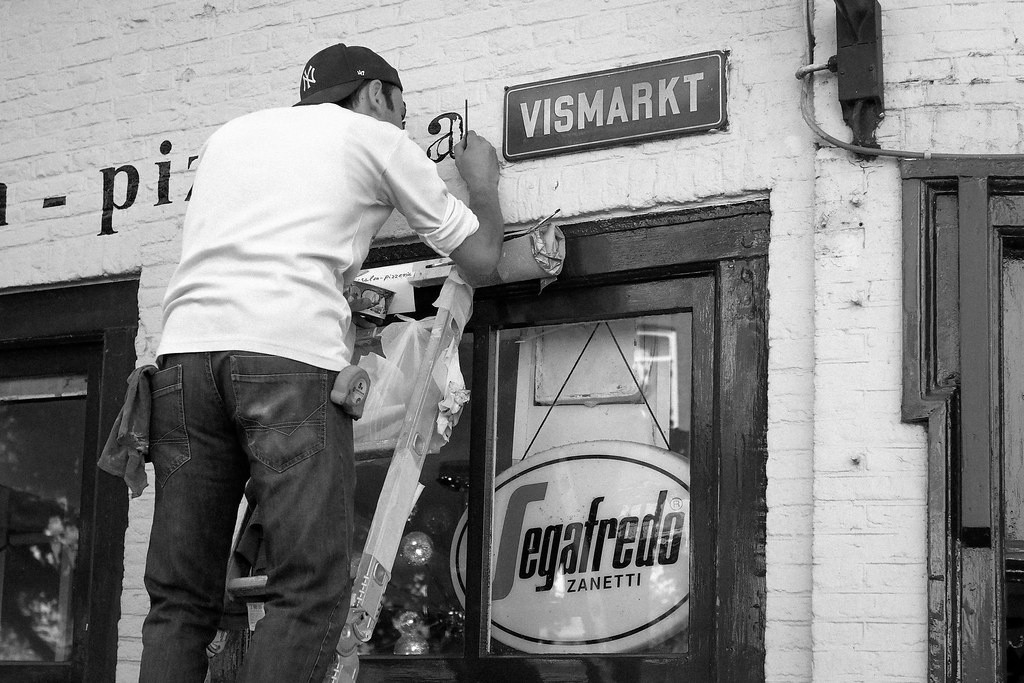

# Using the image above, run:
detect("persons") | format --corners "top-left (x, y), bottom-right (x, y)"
top-left (139, 44), bottom-right (505, 683)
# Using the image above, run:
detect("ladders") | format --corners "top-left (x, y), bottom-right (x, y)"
top-left (223, 264), bottom-right (469, 683)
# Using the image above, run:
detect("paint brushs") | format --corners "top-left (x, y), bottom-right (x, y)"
top-left (464, 98), bottom-right (468, 149)
top-left (525, 209), bottom-right (561, 234)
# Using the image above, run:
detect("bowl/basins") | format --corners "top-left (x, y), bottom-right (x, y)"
top-left (347, 281), bottom-right (396, 319)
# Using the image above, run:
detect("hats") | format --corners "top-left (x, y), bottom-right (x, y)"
top-left (291, 43), bottom-right (404, 107)
